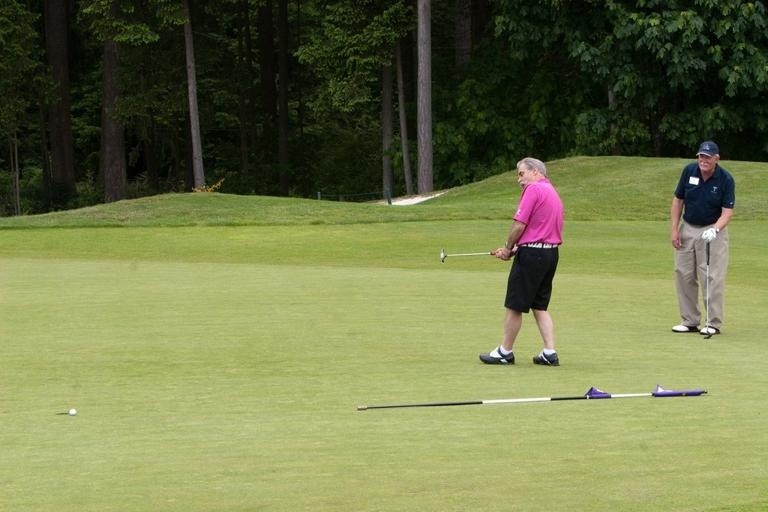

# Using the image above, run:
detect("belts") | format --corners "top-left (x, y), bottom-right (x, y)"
top-left (521, 242), bottom-right (558, 248)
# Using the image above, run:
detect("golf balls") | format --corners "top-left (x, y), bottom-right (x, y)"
top-left (69, 409), bottom-right (77, 416)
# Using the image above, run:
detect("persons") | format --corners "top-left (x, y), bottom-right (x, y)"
top-left (671, 140), bottom-right (734, 335)
top-left (478, 156), bottom-right (564, 366)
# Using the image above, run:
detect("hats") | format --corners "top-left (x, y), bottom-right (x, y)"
top-left (696, 141), bottom-right (720, 157)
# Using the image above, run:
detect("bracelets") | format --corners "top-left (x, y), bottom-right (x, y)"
top-left (714, 226), bottom-right (720, 235)
top-left (505, 242), bottom-right (514, 249)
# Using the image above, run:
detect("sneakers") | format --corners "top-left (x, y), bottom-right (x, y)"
top-left (479, 345), bottom-right (515, 365)
top-left (671, 324), bottom-right (720, 335)
top-left (532, 349), bottom-right (560, 366)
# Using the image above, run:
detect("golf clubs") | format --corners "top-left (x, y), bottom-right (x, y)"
top-left (441, 249), bottom-right (496, 263)
top-left (704, 242), bottom-right (713, 340)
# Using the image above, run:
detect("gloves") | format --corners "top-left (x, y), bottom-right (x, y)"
top-left (702, 228), bottom-right (717, 243)
